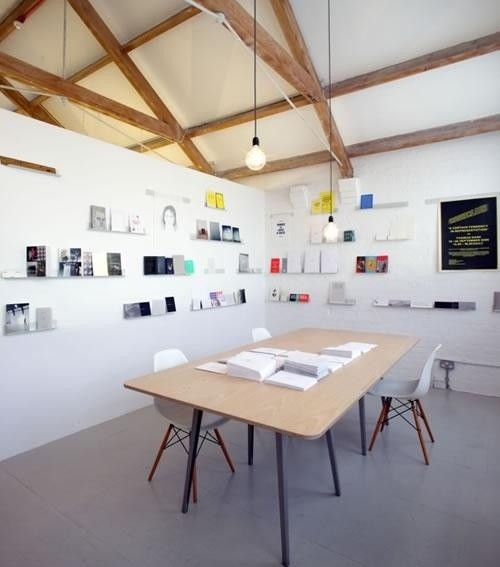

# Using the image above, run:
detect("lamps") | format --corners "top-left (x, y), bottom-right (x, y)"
top-left (316, 1), bottom-right (348, 244)
top-left (242, 1), bottom-right (274, 172)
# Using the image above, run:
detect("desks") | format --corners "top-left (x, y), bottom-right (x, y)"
top-left (121, 326), bottom-right (424, 566)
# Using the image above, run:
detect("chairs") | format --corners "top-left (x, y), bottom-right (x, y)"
top-left (251, 325), bottom-right (272, 342)
top-left (146, 345), bottom-right (237, 505)
top-left (363, 341), bottom-right (444, 467)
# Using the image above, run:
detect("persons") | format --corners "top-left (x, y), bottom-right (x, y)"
top-left (159, 204), bottom-right (178, 232)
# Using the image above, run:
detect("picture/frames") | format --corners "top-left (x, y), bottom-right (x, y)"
top-left (437, 192), bottom-right (499, 272)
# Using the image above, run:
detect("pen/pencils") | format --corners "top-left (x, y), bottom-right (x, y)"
top-left (218, 361), bottom-right (229, 365)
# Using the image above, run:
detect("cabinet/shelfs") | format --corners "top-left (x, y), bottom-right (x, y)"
top-left (276, 236), bottom-right (477, 310)
top-left (0, 221), bottom-right (259, 335)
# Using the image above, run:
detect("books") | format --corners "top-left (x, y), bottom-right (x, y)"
top-left (194, 339), bottom-right (380, 392)
top-left (91, 206), bottom-right (146, 234)
top-left (189, 175), bottom-right (374, 241)
